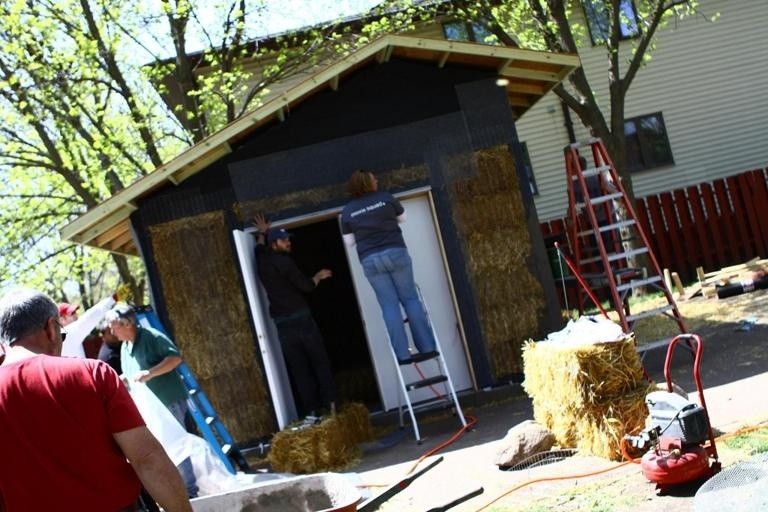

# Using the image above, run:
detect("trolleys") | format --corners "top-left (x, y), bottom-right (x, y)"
top-left (642, 326), bottom-right (723, 499)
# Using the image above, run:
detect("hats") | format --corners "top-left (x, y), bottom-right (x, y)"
top-left (57, 302), bottom-right (81, 317)
top-left (266, 226), bottom-right (292, 246)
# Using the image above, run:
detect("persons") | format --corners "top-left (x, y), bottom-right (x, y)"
top-left (105, 303), bottom-right (189, 434)
top-left (340, 168), bottom-right (435, 364)
top-left (94, 318), bottom-right (123, 377)
top-left (565, 156), bottom-right (622, 274)
top-left (1, 284), bottom-right (194, 511)
top-left (252, 211), bottom-right (334, 417)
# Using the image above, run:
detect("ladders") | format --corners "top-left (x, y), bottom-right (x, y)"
top-left (132, 305), bottom-right (250, 476)
top-left (564, 135), bottom-right (703, 383)
top-left (380, 282), bottom-right (472, 446)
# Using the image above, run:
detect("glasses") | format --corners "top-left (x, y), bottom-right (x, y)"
top-left (54, 318), bottom-right (68, 342)
